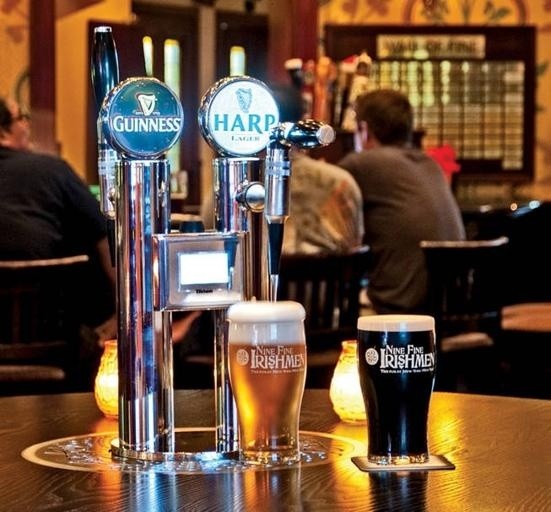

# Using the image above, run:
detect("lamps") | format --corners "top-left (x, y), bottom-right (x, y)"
top-left (329, 338), bottom-right (366, 424)
top-left (93, 340), bottom-right (118, 423)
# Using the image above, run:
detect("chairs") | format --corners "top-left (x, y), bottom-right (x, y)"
top-left (276, 245), bottom-right (373, 389)
top-left (419, 238), bottom-right (510, 392)
top-left (0, 254), bottom-right (90, 393)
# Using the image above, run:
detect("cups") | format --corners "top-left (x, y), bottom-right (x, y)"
top-left (355, 313), bottom-right (440, 471)
top-left (223, 299), bottom-right (309, 459)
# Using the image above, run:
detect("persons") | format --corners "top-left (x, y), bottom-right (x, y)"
top-left (333, 87), bottom-right (477, 341)
top-left (179, 76), bottom-right (366, 362)
top-left (0, 94), bottom-right (119, 396)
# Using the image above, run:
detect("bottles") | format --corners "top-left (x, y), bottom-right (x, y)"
top-left (93, 339), bottom-right (121, 419)
top-left (326, 341), bottom-right (369, 425)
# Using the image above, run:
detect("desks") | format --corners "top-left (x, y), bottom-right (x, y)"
top-left (0, 390), bottom-right (550, 512)
